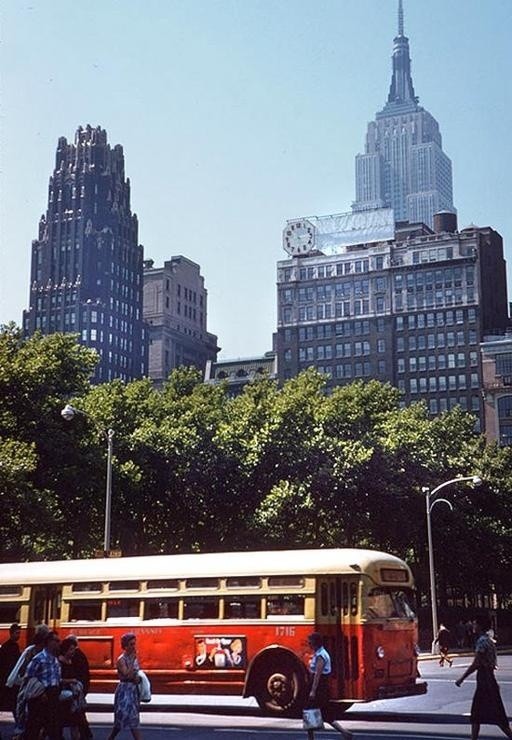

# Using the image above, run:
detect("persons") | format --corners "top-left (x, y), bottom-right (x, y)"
top-left (455, 619), bottom-right (496, 650)
top-left (0, 624), bottom-right (94, 740)
top-left (434, 624), bottom-right (453, 667)
top-left (302, 631), bottom-right (353, 740)
top-left (455, 616), bottom-right (512, 740)
top-left (107, 633), bottom-right (142, 740)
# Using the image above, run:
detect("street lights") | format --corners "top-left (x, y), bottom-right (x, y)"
top-left (61, 404), bottom-right (113, 551)
top-left (426, 476), bottom-right (483, 655)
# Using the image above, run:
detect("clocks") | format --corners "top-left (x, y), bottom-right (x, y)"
top-left (282, 220), bottom-right (316, 259)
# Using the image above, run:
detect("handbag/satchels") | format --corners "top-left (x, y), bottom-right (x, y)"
top-left (302, 708), bottom-right (324, 731)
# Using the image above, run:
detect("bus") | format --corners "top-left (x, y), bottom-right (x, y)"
top-left (0, 548), bottom-right (428, 718)
